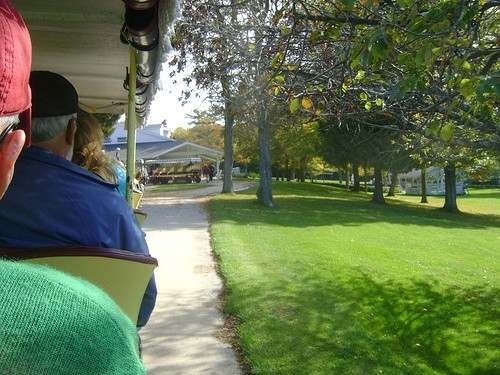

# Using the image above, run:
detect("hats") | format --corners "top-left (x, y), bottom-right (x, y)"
top-left (29, 70), bottom-right (78, 118)
top-left (0, 0), bottom-right (32, 116)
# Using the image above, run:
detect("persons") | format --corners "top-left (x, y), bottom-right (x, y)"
top-left (0, 0), bottom-right (158, 375)
top-left (202, 160), bottom-right (215, 182)
